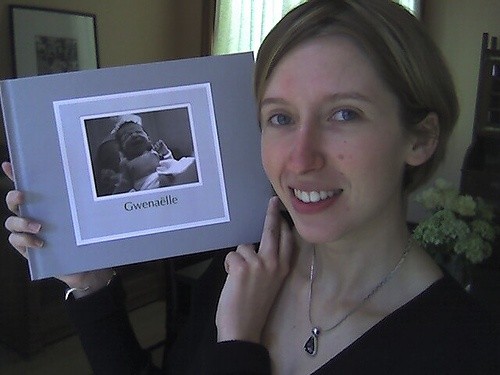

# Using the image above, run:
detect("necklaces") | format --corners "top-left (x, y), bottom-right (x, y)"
top-left (302, 228), bottom-right (417, 357)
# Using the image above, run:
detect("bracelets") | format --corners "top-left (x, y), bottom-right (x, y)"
top-left (65, 270), bottom-right (118, 301)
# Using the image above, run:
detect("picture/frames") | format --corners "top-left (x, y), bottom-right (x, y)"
top-left (7, 3), bottom-right (101, 81)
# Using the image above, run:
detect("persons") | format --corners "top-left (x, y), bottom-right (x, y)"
top-left (97, 120), bottom-right (183, 197)
top-left (1, 0), bottom-right (500, 375)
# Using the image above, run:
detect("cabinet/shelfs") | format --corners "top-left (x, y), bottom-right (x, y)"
top-left (1, 186), bottom-right (169, 362)
